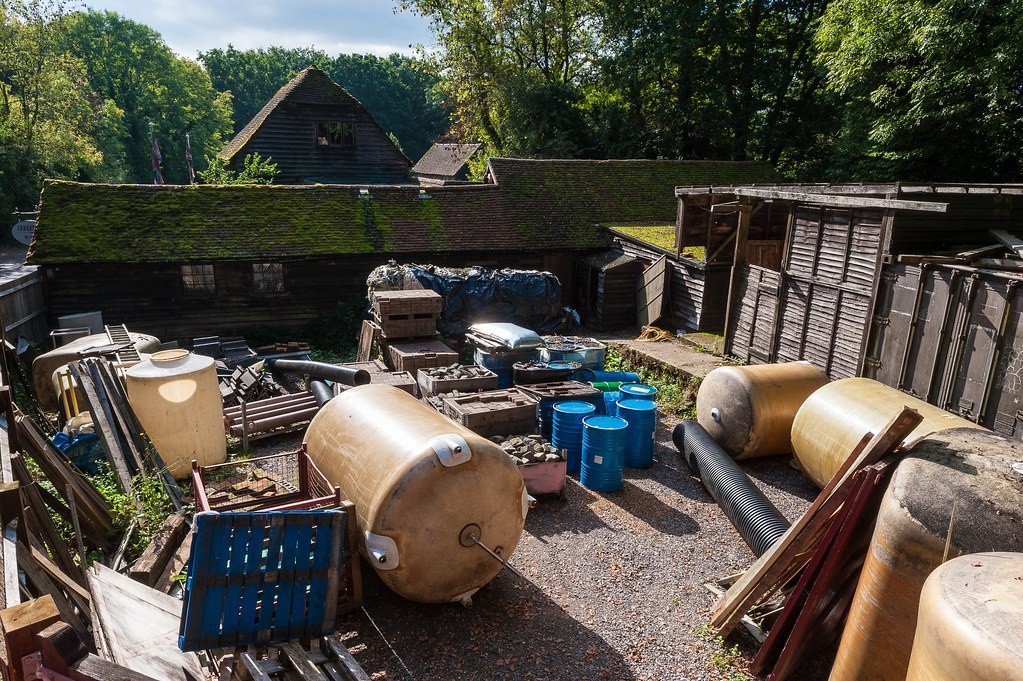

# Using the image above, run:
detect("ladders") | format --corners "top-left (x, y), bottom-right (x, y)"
top-left (104, 322), bottom-right (145, 384)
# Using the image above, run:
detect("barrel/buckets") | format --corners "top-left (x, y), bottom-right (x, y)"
top-left (551, 400), bottom-right (596, 473)
top-left (512, 358), bottom-right (583, 380)
top-left (695, 359), bottom-right (831, 463)
top-left (602, 391), bottom-right (624, 415)
top-left (905, 552), bottom-right (1023, 681)
top-left (616, 398), bottom-right (657, 469)
top-left (619, 382), bottom-right (658, 402)
top-left (581, 415), bottom-right (629, 492)
top-left (827, 428), bottom-right (1023, 681)
top-left (584, 381), bottom-right (640, 392)
top-left (574, 368), bottom-right (642, 382)
top-left (790, 376), bottom-right (995, 494)
top-left (300, 382), bottom-right (528, 603)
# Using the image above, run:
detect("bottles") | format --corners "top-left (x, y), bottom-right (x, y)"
top-left (126, 347), bottom-right (228, 481)
